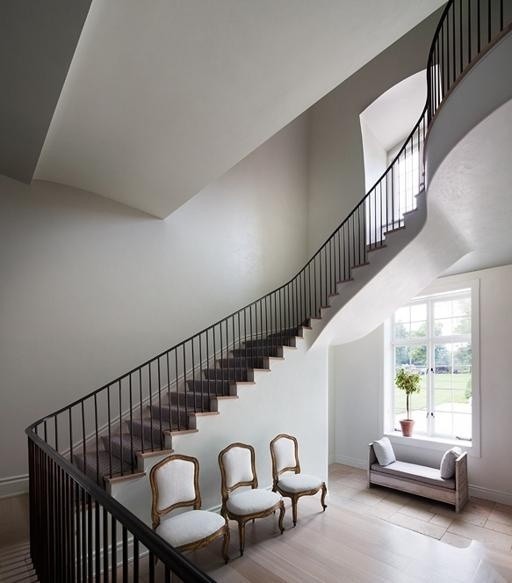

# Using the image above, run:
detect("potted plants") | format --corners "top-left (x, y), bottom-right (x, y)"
top-left (394, 364), bottom-right (423, 436)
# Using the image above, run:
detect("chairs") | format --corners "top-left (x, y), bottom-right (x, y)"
top-left (218, 442), bottom-right (286, 556)
top-left (149, 453), bottom-right (230, 582)
top-left (270, 433), bottom-right (328, 526)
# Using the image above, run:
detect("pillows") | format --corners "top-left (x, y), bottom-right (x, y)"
top-left (440, 446), bottom-right (462, 478)
top-left (372, 436), bottom-right (396, 465)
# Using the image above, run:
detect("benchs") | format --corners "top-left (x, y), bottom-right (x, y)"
top-left (367, 440), bottom-right (469, 513)
